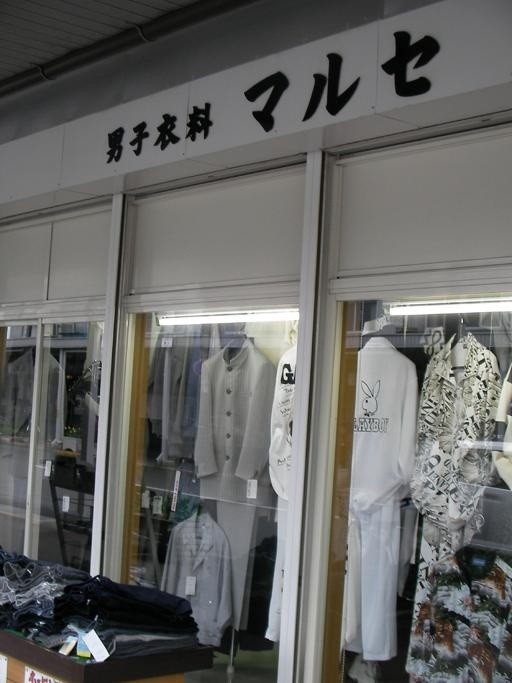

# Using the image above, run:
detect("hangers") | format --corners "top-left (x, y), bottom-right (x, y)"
top-left (227, 323), bottom-right (247, 361)
top-left (194, 499), bottom-right (205, 541)
top-left (32, 344), bottom-right (36, 367)
top-left (450, 313), bottom-right (467, 345)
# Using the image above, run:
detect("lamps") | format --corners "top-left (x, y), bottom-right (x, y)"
top-left (154, 307), bottom-right (304, 326)
top-left (386, 300), bottom-right (512, 318)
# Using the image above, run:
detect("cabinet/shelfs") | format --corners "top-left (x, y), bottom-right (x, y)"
top-left (50, 471), bottom-right (161, 590)
top-left (0, 630), bottom-right (217, 683)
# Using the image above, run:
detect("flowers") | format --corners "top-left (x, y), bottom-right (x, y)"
top-left (62, 424), bottom-right (83, 436)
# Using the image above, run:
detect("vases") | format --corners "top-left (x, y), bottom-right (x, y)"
top-left (63, 436), bottom-right (84, 454)
